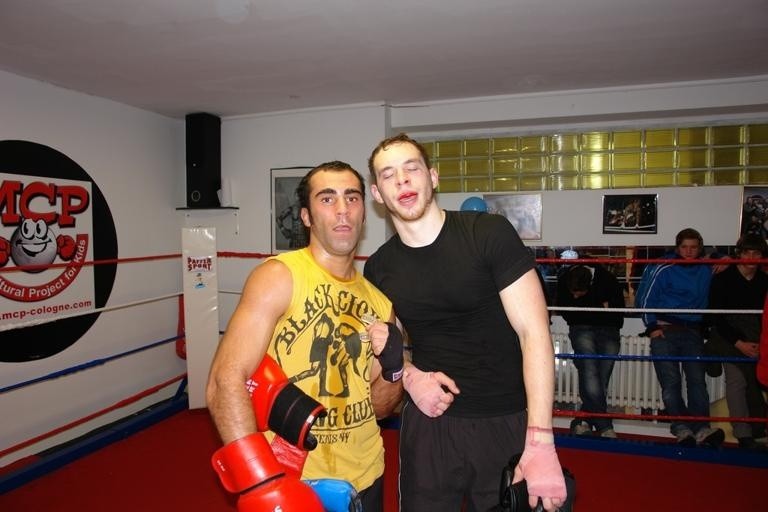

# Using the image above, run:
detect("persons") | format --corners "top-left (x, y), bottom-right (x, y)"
top-left (203, 159), bottom-right (405, 511)
top-left (634, 227), bottom-right (733, 448)
top-left (743, 196), bottom-right (768, 231)
top-left (276, 189), bottom-right (306, 250)
top-left (707, 233), bottom-right (768, 447)
top-left (556, 263), bottom-right (626, 438)
top-left (538, 248), bottom-right (557, 275)
top-left (360, 130), bottom-right (569, 511)
top-left (609, 195), bottom-right (642, 226)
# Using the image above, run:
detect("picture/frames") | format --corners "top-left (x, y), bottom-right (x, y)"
top-left (481, 193), bottom-right (543, 240)
top-left (270, 166), bottom-right (315, 256)
top-left (739, 185), bottom-right (768, 244)
top-left (603, 193), bottom-right (658, 234)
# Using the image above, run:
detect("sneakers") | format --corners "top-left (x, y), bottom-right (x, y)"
top-left (694, 425), bottom-right (728, 447)
top-left (570, 418), bottom-right (596, 439)
top-left (599, 427), bottom-right (622, 442)
top-left (737, 436), bottom-right (758, 450)
top-left (673, 427), bottom-right (698, 449)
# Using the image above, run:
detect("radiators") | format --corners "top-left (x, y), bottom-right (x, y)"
top-left (543, 332), bottom-right (728, 411)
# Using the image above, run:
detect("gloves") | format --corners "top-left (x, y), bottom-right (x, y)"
top-left (370, 319), bottom-right (408, 386)
top-left (210, 427), bottom-right (326, 512)
top-left (400, 362), bottom-right (447, 420)
top-left (515, 424), bottom-right (571, 504)
top-left (243, 349), bottom-right (329, 483)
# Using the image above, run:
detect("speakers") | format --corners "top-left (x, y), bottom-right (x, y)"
top-left (186, 113), bottom-right (221, 208)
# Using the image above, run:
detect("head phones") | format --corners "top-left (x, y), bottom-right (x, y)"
top-left (734, 233), bottom-right (768, 255)
